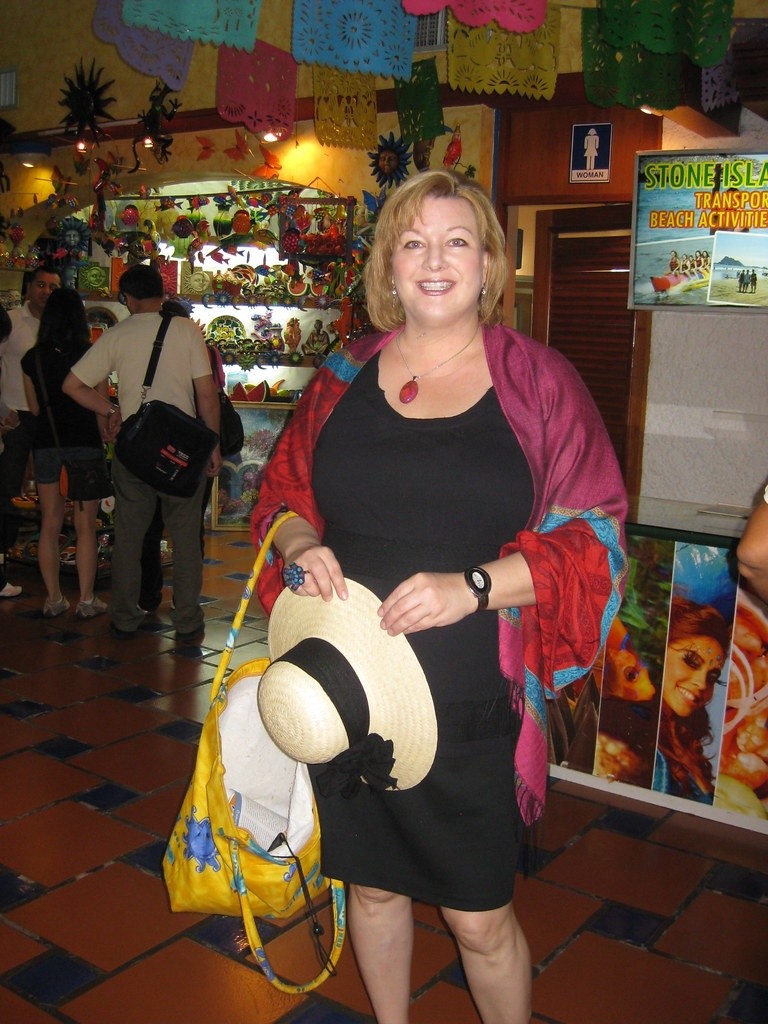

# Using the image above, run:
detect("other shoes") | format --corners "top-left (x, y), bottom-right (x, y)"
top-left (137, 603), bottom-right (157, 613)
top-left (110, 622), bottom-right (135, 639)
top-left (0, 582), bottom-right (22, 597)
top-left (180, 622), bottom-right (206, 645)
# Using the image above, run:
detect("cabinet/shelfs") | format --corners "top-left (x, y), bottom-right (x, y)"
top-left (2, 288), bottom-right (350, 588)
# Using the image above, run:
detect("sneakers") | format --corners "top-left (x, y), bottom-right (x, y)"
top-left (75, 595), bottom-right (108, 620)
top-left (43, 593), bottom-right (71, 618)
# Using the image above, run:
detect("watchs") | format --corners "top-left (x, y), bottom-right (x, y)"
top-left (464, 567), bottom-right (491, 611)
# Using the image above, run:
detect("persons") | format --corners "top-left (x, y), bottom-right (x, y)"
top-left (736, 485), bottom-right (768, 606)
top-left (137, 301), bottom-right (226, 615)
top-left (664, 249), bottom-right (711, 283)
top-left (602, 595), bottom-right (729, 805)
top-left (737, 269), bottom-right (757, 294)
top-left (62, 265), bottom-right (223, 634)
top-left (0, 266), bottom-right (113, 619)
top-left (252, 172), bottom-right (628, 1024)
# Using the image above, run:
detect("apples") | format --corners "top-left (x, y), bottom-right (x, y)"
top-left (272, 388), bottom-right (296, 396)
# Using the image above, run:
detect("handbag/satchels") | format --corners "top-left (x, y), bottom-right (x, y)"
top-left (114, 399), bottom-right (220, 496)
top-left (63, 445), bottom-right (114, 502)
top-left (159, 509), bottom-right (346, 994)
top-left (206, 344), bottom-right (244, 455)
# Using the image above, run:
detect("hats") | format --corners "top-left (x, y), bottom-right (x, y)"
top-left (256, 574), bottom-right (439, 791)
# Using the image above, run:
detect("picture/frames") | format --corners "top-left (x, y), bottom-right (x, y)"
top-left (210, 401), bottom-right (298, 531)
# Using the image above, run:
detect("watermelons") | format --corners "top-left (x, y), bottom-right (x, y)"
top-left (289, 281), bottom-right (332, 297)
top-left (230, 380), bottom-right (270, 401)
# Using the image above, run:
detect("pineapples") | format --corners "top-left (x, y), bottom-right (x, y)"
top-left (217, 489), bottom-right (238, 515)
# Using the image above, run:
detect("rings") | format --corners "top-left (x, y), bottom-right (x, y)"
top-left (282, 562), bottom-right (310, 591)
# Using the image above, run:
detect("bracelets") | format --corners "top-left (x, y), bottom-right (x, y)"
top-left (107, 404), bottom-right (119, 419)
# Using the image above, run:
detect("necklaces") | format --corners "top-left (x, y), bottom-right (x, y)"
top-left (396, 327), bottom-right (478, 404)
